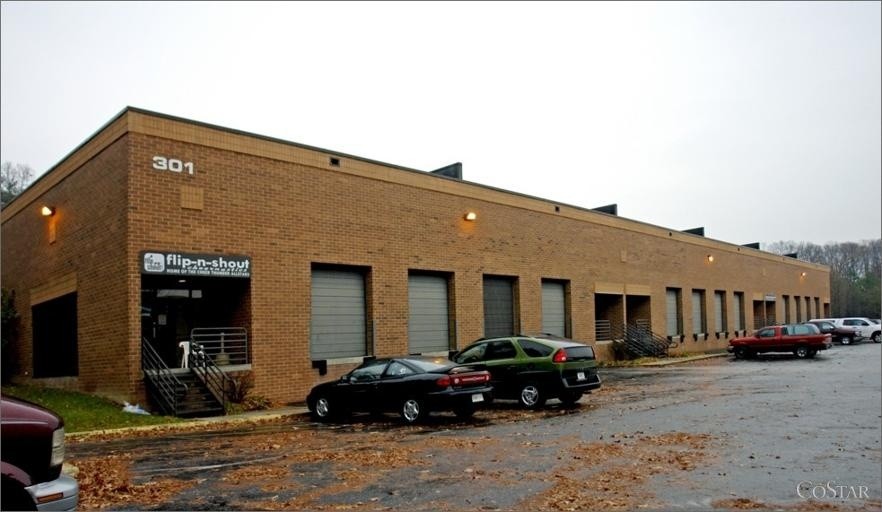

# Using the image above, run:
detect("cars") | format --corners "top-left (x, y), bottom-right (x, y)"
top-left (801, 321), bottom-right (862, 345)
top-left (307, 355), bottom-right (496, 426)
top-left (1, 390), bottom-right (81, 512)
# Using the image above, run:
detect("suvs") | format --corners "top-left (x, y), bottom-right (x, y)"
top-left (450, 331), bottom-right (602, 410)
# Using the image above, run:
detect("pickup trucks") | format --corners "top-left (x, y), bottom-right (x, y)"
top-left (727, 323), bottom-right (832, 359)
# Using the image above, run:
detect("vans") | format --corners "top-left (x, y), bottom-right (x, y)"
top-left (808, 317), bottom-right (881, 343)
